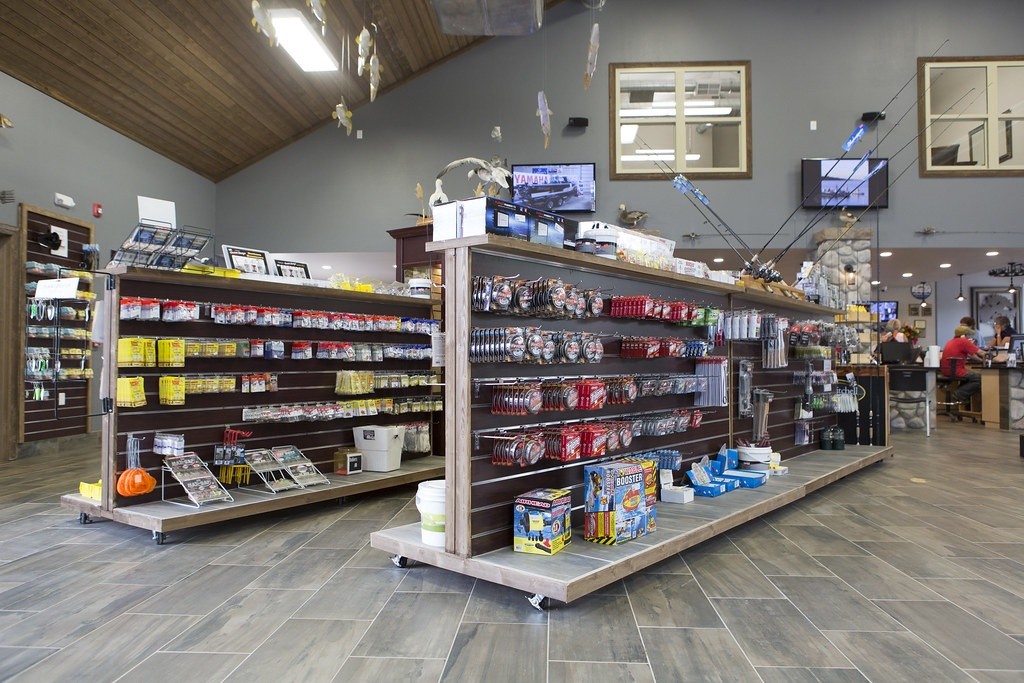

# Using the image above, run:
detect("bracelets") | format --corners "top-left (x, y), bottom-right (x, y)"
top-left (997, 335), bottom-right (999, 336)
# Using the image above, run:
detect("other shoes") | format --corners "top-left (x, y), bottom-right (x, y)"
top-left (952, 392), bottom-right (969, 404)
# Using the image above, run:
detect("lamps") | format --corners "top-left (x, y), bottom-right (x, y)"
top-left (260, 7), bottom-right (339, 73)
top-left (955, 274), bottom-right (967, 302)
top-left (920, 281), bottom-right (927, 306)
top-left (1006, 263), bottom-right (1018, 293)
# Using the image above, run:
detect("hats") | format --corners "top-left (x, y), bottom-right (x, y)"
top-left (955, 326), bottom-right (975, 336)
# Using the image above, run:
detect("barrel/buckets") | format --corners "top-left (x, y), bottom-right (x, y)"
top-left (415, 479), bottom-right (446, 548)
top-left (409, 278), bottom-right (431, 299)
top-left (737, 444), bottom-right (773, 479)
top-left (575, 228), bottom-right (619, 260)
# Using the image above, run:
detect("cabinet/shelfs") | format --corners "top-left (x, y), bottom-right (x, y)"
top-left (369, 232), bottom-right (895, 613)
top-left (59, 266), bottom-right (445, 544)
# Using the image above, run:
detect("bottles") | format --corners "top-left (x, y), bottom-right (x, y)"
top-left (822, 426), bottom-right (845, 450)
top-left (1007, 351), bottom-right (1016, 367)
top-left (835, 304), bottom-right (871, 366)
top-left (333, 446), bottom-right (363, 475)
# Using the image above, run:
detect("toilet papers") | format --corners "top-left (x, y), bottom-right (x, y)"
top-left (924, 346), bottom-right (940, 367)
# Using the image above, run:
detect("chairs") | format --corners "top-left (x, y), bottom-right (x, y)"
top-left (936, 357), bottom-right (978, 424)
top-left (889, 369), bottom-right (931, 436)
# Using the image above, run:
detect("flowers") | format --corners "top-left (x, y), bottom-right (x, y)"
top-left (900, 323), bottom-right (921, 346)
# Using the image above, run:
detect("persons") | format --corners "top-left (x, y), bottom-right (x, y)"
top-left (882, 318), bottom-right (909, 343)
top-left (960, 316), bottom-right (987, 348)
top-left (942, 325), bottom-right (988, 400)
top-left (987, 315), bottom-right (1018, 348)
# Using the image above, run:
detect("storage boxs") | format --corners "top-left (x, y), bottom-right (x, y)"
top-left (769, 466), bottom-right (788, 476)
top-left (513, 488), bottom-right (572, 556)
top-left (687, 449), bottom-right (766, 497)
top-left (584, 459), bottom-right (658, 546)
top-left (660, 469), bottom-right (694, 504)
top-left (433, 196), bottom-right (578, 252)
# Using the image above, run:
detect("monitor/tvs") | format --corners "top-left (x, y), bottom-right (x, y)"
top-left (801, 158), bottom-right (889, 209)
top-left (510, 162), bottom-right (596, 213)
top-left (852, 300), bottom-right (898, 322)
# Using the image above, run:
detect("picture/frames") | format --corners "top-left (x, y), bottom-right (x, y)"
top-left (968, 109), bottom-right (1012, 166)
top-left (970, 287), bottom-right (1021, 346)
